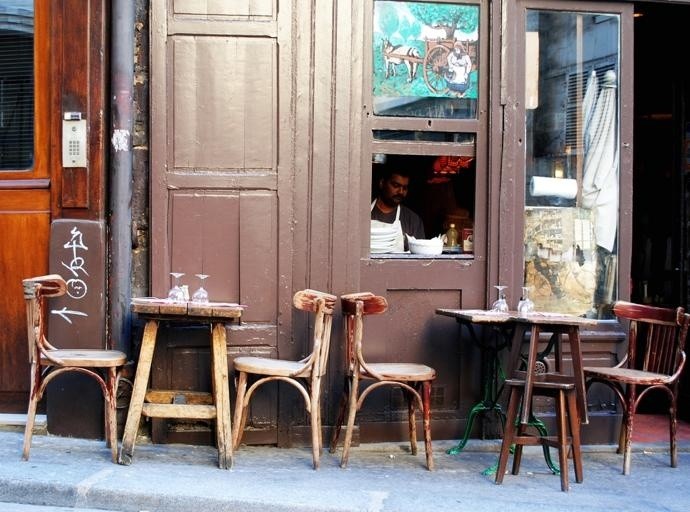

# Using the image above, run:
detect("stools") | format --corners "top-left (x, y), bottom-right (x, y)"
top-left (494, 382), bottom-right (583, 490)
top-left (501, 313), bottom-right (595, 426)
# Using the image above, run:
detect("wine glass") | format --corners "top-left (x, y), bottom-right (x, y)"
top-left (166, 272), bottom-right (186, 302)
top-left (190, 273), bottom-right (212, 303)
top-left (491, 285), bottom-right (510, 313)
top-left (516, 285), bottom-right (535, 314)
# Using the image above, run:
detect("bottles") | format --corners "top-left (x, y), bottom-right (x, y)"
top-left (446, 223), bottom-right (458, 247)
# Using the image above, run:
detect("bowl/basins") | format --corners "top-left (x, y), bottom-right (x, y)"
top-left (408, 238), bottom-right (444, 255)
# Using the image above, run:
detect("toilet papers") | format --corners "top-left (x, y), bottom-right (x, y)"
top-left (529, 176), bottom-right (578, 200)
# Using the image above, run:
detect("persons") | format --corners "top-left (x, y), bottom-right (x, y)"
top-left (446, 39), bottom-right (472, 99)
top-left (370, 163), bottom-right (427, 254)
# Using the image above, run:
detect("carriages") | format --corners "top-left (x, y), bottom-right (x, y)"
top-left (381, 33), bottom-right (479, 97)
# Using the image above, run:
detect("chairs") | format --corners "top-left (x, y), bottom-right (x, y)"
top-left (231, 289), bottom-right (337, 469)
top-left (565, 301), bottom-right (690, 478)
top-left (330, 292), bottom-right (438, 470)
top-left (20, 274), bottom-right (126, 465)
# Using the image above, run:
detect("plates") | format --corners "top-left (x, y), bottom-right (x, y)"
top-left (370, 219), bottom-right (399, 255)
